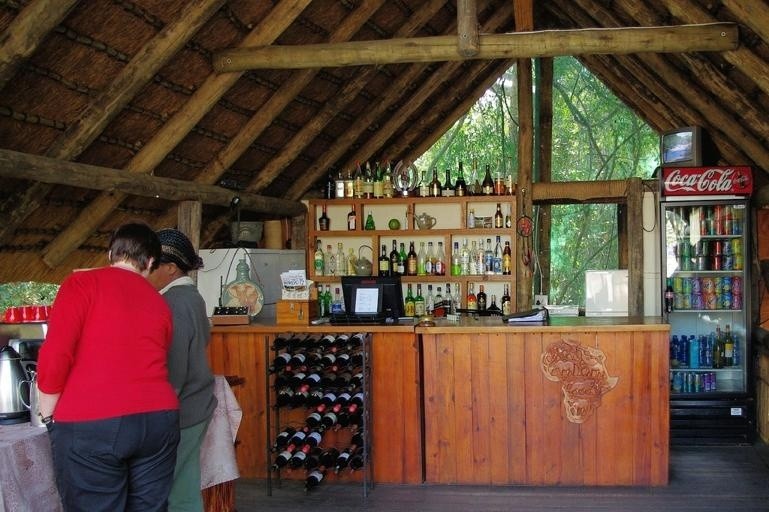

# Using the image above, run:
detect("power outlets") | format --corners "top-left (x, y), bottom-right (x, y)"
top-left (535, 295), bottom-right (548, 305)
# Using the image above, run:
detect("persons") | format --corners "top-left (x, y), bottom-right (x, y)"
top-left (147, 228), bottom-right (219, 511)
top-left (36, 223), bottom-right (180, 511)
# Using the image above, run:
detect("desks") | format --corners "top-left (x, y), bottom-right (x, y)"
top-left (0, 374), bottom-right (245, 512)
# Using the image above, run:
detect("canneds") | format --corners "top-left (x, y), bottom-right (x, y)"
top-left (666, 206), bottom-right (743, 235)
top-left (665, 277), bottom-right (741, 310)
top-left (666, 239), bottom-right (744, 270)
top-left (672, 372), bottom-right (716, 392)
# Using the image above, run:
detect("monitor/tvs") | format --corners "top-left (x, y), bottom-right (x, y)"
top-left (660, 126), bottom-right (720, 168)
top-left (341, 273), bottom-right (406, 319)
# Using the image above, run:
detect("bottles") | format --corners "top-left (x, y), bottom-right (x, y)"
top-left (266, 332), bottom-right (370, 492)
top-left (665, 286), bottom-right (674, 313)
top-left (314, 157), bottom-right (515, 317)
top-left (671, 324), bottom-right (739, 369)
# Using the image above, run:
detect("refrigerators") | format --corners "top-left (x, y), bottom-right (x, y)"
top-left (654, 165), bottom-right (755, 448)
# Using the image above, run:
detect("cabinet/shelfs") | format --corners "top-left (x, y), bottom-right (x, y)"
top-left (265, 333), bottom-right (374, 498)
top-left (308, 196), bottom-right (516, 317)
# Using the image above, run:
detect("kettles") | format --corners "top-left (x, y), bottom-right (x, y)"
top-left (0, 345), bottom-right (37, 425)
top-left (18, 371), bottom-right (46, 427)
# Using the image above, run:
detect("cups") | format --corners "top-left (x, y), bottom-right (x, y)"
top-left (5, 305), bottom-right (53, 323)
